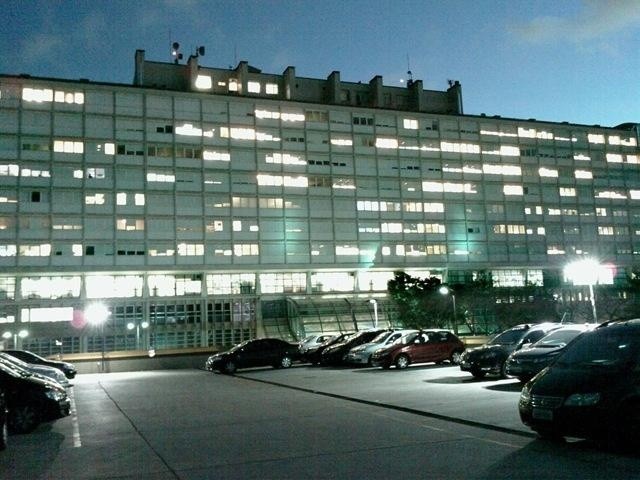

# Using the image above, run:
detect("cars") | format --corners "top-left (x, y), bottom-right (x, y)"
top-left (301, 328), bottom-right (465, 368)
top-left (460, 318), bottom-right (639, 453)
top-left (206, 338), bottom-right (300, 375)
top-left (0, 348), bottom-right (77, 449)
top-left (578, 263), bottom-right (598, 324)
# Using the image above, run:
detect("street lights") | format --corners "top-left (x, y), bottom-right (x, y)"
top-left (441, 287), bottom-right (458, 334)
top-left (128, 321), bottom-right (148, 350)
top-left (92, 308), bottom-right (107, 372)
top-left (3, 330), bottom-right (28, 350)
top-left (370, 299), bottom-right (378, 328)
